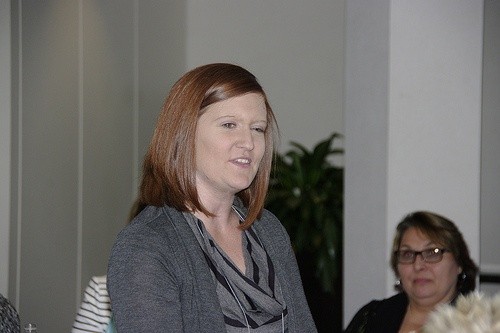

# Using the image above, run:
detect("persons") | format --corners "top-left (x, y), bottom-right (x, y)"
top-left (343, 212), bottom-right (479, 333)
top-left (103, 64), bottom-right (319, 333)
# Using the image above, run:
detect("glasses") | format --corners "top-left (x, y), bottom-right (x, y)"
top-left (395, 247), bottom-right (453, 265)
